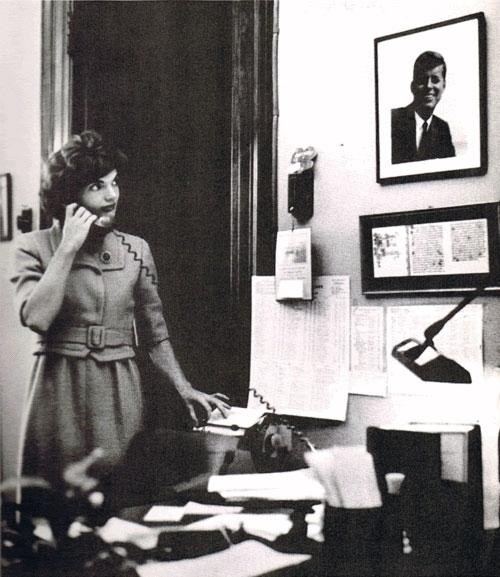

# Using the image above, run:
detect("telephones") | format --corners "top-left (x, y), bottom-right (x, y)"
top-left (60, 189), bottom-right (112, 234)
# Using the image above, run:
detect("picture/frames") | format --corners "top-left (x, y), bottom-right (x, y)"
top-left (0, 172), bottom-right (13, 242)
top-left (358, 202), bottom-right (500, 299)
top-left (375, 10), bottom-right (486, 183)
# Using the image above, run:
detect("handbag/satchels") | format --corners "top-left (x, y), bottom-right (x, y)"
top-left (128, 428), bottom-right (210, 490)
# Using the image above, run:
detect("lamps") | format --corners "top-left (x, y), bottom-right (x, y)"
top-left (390, 267), bottom-right (500, 384)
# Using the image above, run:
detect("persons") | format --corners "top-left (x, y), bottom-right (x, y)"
top-left (10, 130), bottom-right (231, 477)
top-left (392, 51), bottom-right (455, 165)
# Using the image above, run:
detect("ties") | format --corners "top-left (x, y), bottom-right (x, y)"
top-left (419, 122), bottom-right (428, 153)
